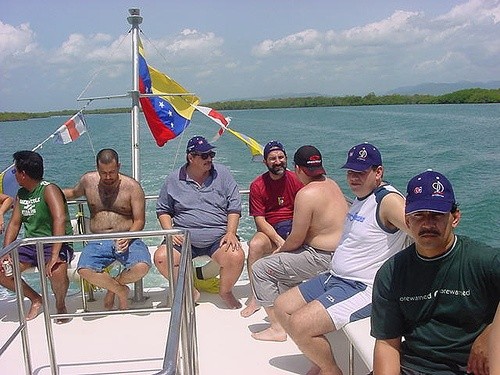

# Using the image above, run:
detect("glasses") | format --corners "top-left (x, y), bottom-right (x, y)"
top-left (189, 152), bottom-right (215, 160)
top-left (12, 170), bottom-right (28, 175)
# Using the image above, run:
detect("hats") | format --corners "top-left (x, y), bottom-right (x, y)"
top-left (339, 142), bottom-right (382, 172)
top-left (294, 145), bottom-right (326, 176)
top-left (405, 170), bottom-right (455, 216)
top-left (187, 136), bottom-right (217, 152)
top-left (264, 141), bottom-right (286, 160)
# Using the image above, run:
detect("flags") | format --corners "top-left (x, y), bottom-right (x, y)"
top-left (228, 128), bottom-right (265, 158)
top-left (0, 169), bottom-right (23, 202)
top-left (52, 110), bottom-right (89, 144)
top-left (136, 33), bottom-right (201, 148)
top-left (194, 106), bottom-right (232, 143)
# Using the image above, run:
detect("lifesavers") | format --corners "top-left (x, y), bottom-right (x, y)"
top-left (193, 261), bottom-right (221, 280)
top-left (71, 219), bottom-right (84, 234)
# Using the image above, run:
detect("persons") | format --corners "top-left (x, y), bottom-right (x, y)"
top-left (154, 136), bottom-right (245, 309)
top-left (370, 168), bottom-right (500, 375)
top-left (251, 145), bottom-right (348, 342)
top-left (0, 150), bottom-right (74, 324)
top-left (0, 193), bottom-right (14, 235)
top-left (273, 142), bottom-right (460, 375)
top-left (240, 140), bottom-right (306, 317)
top-left (488, 301), bottom-right (500, 375)
top-left (61, 148), bottom-right (151, 311)
